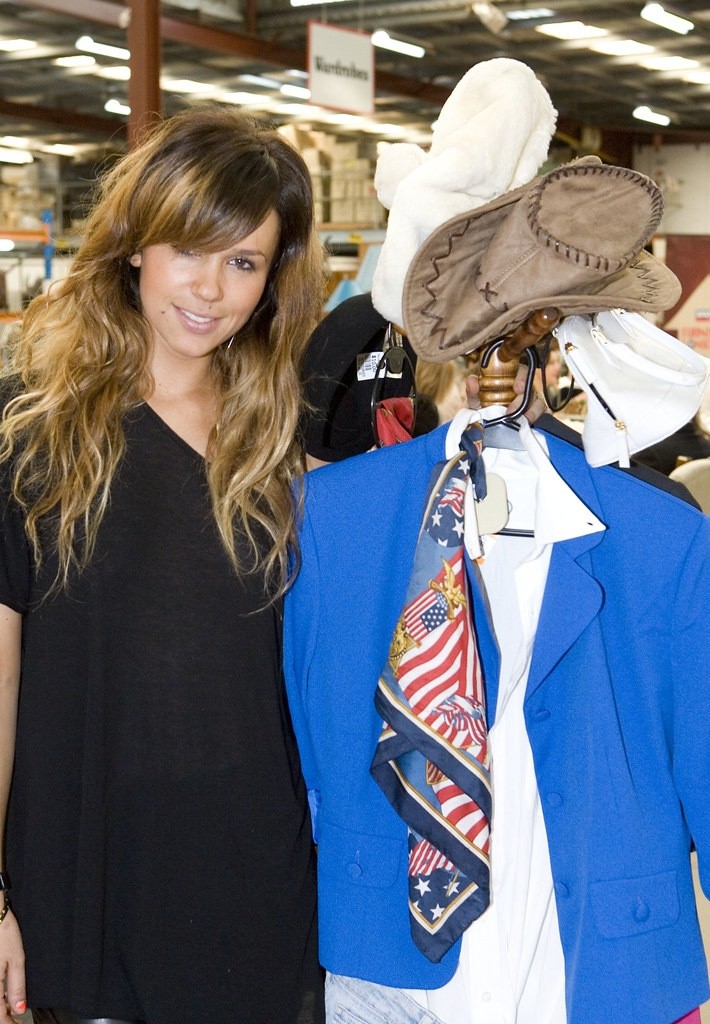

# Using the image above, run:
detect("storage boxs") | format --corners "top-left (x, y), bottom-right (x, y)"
top-left (278, 123), bottom-right (388, 223)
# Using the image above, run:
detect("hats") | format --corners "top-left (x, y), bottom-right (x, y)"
top-left (553, 309), bottom-right (710, 468)
top-left (372, 56), bottom-right (558, 328)
top-left (293, 294), bottom-right (417, 462)
top-left (402, 155), bottom-right (683, 364)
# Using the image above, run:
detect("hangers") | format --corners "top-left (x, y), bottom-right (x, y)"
top-left (477, 335), bottom-right (537, 538)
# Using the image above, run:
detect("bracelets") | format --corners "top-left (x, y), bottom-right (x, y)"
top-left (0, 873), bottom-right (12, 920)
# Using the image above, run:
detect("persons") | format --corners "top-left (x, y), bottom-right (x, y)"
top-left (543, 336), bottom-right (563, 386)
top-left (1, 102), bottom-right (547, 1024)
